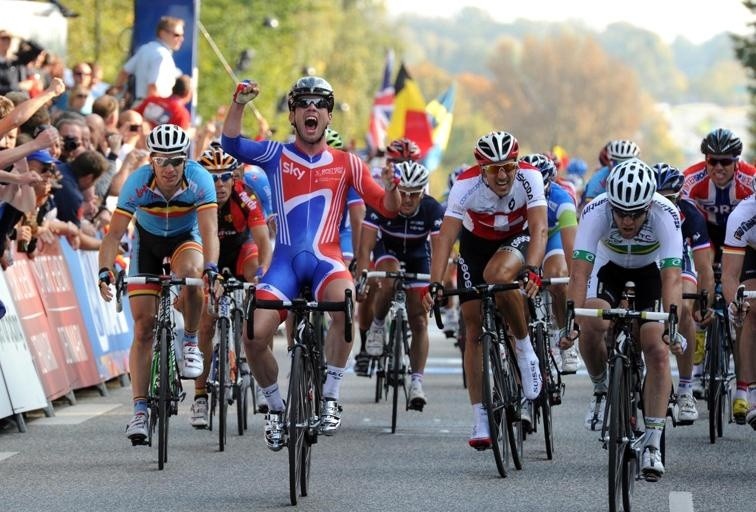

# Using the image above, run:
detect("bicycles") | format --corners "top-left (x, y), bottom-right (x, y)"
top-left (204, 267), bottom-right (269, 438)
top-left (359, 261), bottom-right (446, 434)
top-left (564, 287), bottom-right (678, 512)
top-left (245, 283), bottom-right (356, 507)
top-left (440, 254), bottom-right (571, 480)
top-left (113, 267), bottom-right (224, 472)
top-left (680, 263), bottom-right (756, 444)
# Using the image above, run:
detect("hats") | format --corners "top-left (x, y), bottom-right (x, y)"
top-left (27, 149), bottom-right (61, 165)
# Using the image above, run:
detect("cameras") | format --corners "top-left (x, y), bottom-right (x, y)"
top-left (63, 135), bottom-right (76, 151)
top-left (129, 124), bottom-right (140, 132)
top-left (18, 240), bottom-right (29, 253)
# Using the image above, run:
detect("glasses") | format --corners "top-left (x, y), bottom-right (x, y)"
top-left (483, 161), bottom-right (518, 174)
top-left (613, 208), bottom-right (645, 218)
top-left (152, 155), bottom-right (186, 166)
top-left (292, 98), bottom-right (327, 108)
top-left (708, 158), bottom-right (738, 165)
top-left (399, 189), bottom-right (423, 198)
top-left (664, 192), bottom-right (680, 200)
top-left (212, 172), bottom-right (233, 182)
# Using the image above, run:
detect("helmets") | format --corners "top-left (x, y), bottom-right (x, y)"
top-left (288, 76), bottom-right (334, 99)
top-left (474, 131), bottom-right (557, 184)
top-left (146, 123), bottom-right (242, 171)
top-left (701, 128), bottom-right (742, 155)
top-left (326, 129), bottom-right (342, 149)
top-left (384, 139), bottom-right (428, 187)
top-left (607, 139), bottom-right (684, 212)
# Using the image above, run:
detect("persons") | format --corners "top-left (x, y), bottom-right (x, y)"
top-left (0, 15), bottom-right (227, 273)
top-left (99, 76), bottom-right (755, 481)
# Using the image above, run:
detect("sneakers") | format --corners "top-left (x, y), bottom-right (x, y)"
top-left (469, 345), bottom-right (542, 447)
top-left (642, 445), bottom-right (664, 477)
top-left (732, 398), bottom-right (756, 430)
top-left (127, 340), bottom-right (208, 437)
top-left (264, 397), bottom-right (342, 451)
top-left (354, 321), bottom-right (385, 372)
top-left (677, 392), bottom-right (699, 420)
top-left (409, 384), bottom-right (426, 407)
top-left (585, 391), bottom-right (607, 430)
top-left (560, 344), bottom-right (578, 371)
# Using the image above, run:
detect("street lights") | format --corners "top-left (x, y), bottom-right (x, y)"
top-left (225, 7), bottom-right (279, 78)
